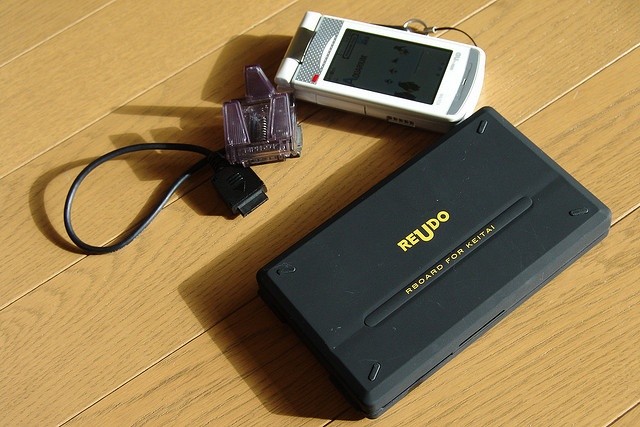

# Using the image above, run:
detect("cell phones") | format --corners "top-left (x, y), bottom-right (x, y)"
top-left (273, 11), bottom-right (486, 134)
